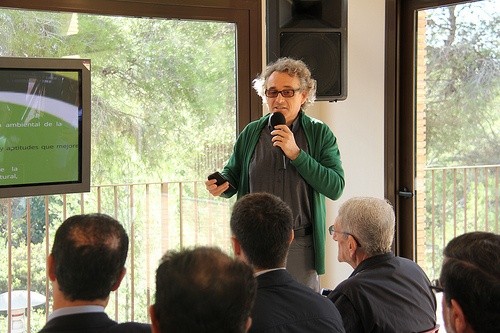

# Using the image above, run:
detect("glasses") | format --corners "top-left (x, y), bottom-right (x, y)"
top-left (329, 225), bottom-right (361, 247)
top-left (428, 280), bottom-right (445, 292)
top-left (265, 88), bottom-right (300, 98)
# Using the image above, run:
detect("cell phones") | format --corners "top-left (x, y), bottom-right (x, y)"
top-left (208, 172), bottom-right (237, 198)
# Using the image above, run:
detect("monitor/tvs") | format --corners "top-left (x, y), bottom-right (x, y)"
top-left (0, 56), bottom-right (92, 198)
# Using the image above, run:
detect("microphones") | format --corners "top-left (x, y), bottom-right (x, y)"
top-left (271, 112), bottom-right (287, 131)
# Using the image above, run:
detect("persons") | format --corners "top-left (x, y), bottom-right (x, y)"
top-left (229, 192), bottom-right (345, 333)
top-left (149, 244), bottom-right (258, 333)
top-left (205, 56), bottom-right (345, 295)
top-left (327, 197), bottom-right (437, 333)
top-left (36, 213), bottom-right (153, 333)
top-left (428, 230), bottom-right (500, 333)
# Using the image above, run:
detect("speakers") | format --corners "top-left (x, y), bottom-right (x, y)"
top-left (265, 0), bottom-right (348, 103)
